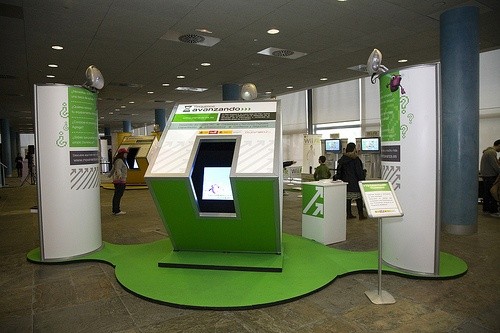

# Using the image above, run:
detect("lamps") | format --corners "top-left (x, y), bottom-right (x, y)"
top-left (240, 82), bottom-right (257, 101)
top-left (81, 64), bottom-right (104, 93)
top-left (367, 48), bottom-right (389, 74)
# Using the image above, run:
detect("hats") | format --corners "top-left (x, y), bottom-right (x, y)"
top-left (118, 147), bottom-right (129, 153)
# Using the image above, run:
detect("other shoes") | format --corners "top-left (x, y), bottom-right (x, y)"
top-left (359, 215), bottom-right (367, 220)
top-left (115, 211), bottom-right (127, 215)
top-left (347, 215), bottom-right (357, 219)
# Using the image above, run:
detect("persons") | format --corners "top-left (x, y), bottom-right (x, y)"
top-left (337, 143), bottom-right (366, 220)
top-left (480, 139), bottom-right (500, 213)
top-left (15, 153), bottom-right (23, 177)
top-left (112, 149), bottom-right (128, 215)
top-left (314, 156), bottom-right (331, 181)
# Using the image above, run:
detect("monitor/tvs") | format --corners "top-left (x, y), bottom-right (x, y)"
top-left (361, 138), bottom-right (379, 151)
top-left (325, 139), bottom-right (340, 151)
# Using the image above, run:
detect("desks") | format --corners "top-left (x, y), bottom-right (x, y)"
top-left (301, 181), bottom-right (349, 245)
